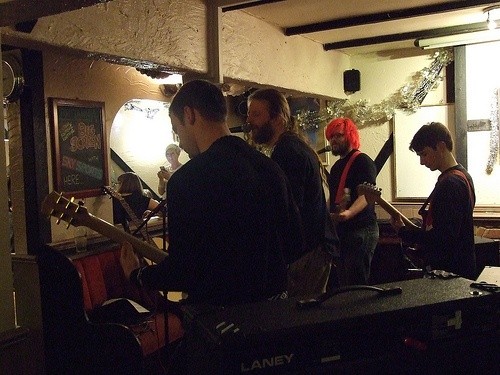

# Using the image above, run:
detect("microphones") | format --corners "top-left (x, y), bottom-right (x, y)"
top-left (229, 123), bottom-right (252, 133)
top-left (317, 145), bottom-right (331, 154)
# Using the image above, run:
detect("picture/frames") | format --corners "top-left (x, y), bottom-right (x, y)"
top-left (51, 97), bottom-right (112, 199)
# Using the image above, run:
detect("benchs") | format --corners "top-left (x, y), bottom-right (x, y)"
top-left (37, 219), bottom-right (187, 375)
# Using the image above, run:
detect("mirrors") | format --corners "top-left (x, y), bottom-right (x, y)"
top-left (108, 98), bottom-right (190, 225)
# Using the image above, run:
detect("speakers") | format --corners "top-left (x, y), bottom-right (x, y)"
top-left (344, 70), bottom-right (360, 92)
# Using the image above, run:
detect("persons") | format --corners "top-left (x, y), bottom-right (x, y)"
top-left (390, 121), bottom-right (476, 280)
top-left (113, 79), bottom-right (379, 313)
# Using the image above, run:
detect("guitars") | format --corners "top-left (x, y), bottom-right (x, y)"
top-left (41, 189), bottom-right (168, 266)
top-left (361, 181), bottom-right (421, 242)
top-left (104, 185), bottom-right (161, 253)
